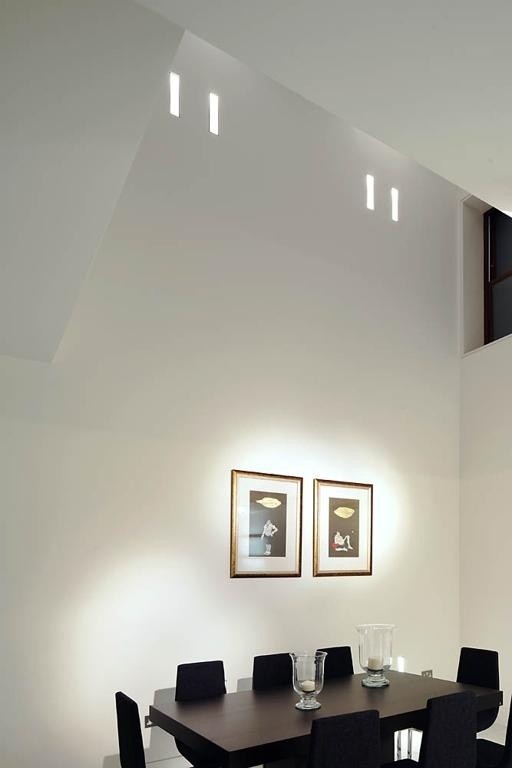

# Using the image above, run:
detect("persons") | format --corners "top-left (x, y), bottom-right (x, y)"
top-left (332, 530), bottom-right (354, 552)
top-left (260, 519), bottom-right (278, 555)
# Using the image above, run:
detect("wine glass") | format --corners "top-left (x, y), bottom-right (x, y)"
top-left (291, 651), bottom-right (327, 711)
top-left (355, 623), bottom-right (394, 688)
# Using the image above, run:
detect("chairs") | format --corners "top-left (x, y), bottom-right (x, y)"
top-left (115, 689), bottom-right (151, 767)
top-left (307, 707), bottom-right (384, 767)
top-left (390, 691), bottom-right (512, 767)
top-left (173, 660), bottom-right (262, 766)
top-left (312, 647), bottom-right (355, 680)
top-left (402, 646), bottom-right (503, 757)
top-left (251, 653), bottom-right (299, 689)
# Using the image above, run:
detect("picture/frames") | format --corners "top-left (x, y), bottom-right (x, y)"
top-left (313, 478), bottom-right (373, 577)
top-left (231, 469), bottom-right (303, 578)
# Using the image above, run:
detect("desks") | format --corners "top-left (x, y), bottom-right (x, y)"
top-left (147, 669), bottom-right (497, 757)
